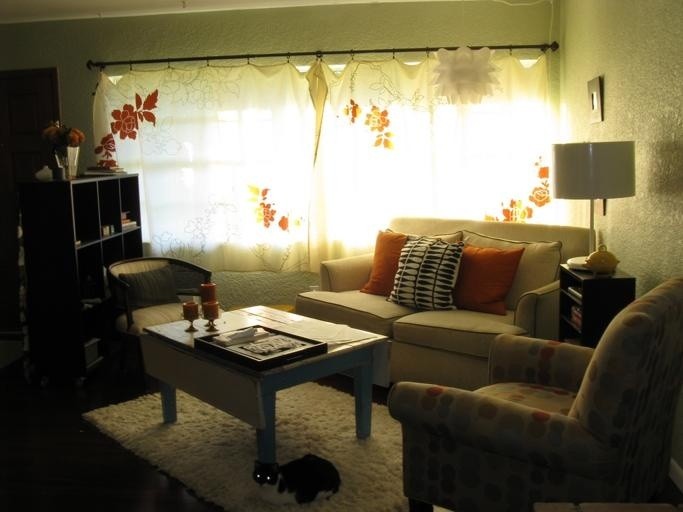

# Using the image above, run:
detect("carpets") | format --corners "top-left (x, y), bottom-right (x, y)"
top-left (82, 381), bottom-right (410, 511)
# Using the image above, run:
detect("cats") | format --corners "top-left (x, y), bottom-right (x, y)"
top-left (254, 453), bottom-right (342, 507)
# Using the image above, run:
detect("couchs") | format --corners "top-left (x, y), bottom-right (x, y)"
top-left (295, 217), bottom-right (595, 389)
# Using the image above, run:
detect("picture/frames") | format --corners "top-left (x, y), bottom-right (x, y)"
top-left (586, 76), bottom-right (602, 124)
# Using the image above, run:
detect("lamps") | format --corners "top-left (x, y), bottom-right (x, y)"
top-left (553, 139), bottom-right (636, 273)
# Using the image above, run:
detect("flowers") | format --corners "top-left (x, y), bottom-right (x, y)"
top-left (46, 121), bottom-right (86, 147)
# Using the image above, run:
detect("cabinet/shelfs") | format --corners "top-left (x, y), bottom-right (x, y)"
top-left (19, 172), bottom-right (143, 404)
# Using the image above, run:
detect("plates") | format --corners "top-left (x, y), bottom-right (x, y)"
top-left (566, 256), bottom-right (592, 271)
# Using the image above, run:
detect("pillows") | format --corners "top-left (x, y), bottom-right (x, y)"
top-left (358, 229), bottom-right (562, 315)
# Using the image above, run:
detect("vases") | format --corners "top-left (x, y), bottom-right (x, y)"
top-left (52, 145), bottom-right (81, 180)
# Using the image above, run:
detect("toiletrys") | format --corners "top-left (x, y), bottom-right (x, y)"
top-left (67, 146), bottom-right (79, 181)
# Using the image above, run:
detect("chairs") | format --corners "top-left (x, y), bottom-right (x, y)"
top-left (106, 256), bottom-right (213, 338)
top-left (386, 275), bottom-right (683, 512)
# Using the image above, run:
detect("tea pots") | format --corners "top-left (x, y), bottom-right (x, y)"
top-left (585, 244), bottom-right (620, 273)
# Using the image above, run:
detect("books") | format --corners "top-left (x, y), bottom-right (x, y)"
top-left (213, 328), bottom-right (269, 347)
top-left (122, 211), bottom-right (136, 227)
